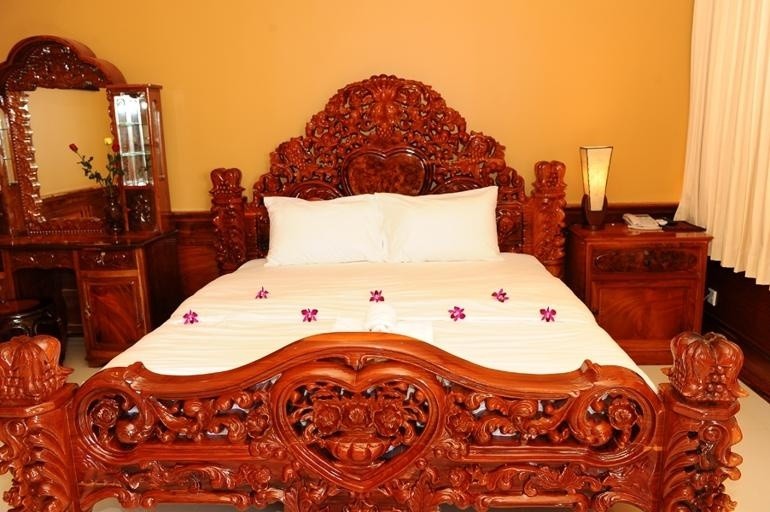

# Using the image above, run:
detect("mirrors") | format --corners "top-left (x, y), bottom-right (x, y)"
top-left (0, 35), bottom-right (172, 231)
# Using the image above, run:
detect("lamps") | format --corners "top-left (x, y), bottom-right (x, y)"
top-left (579, 144), bottom-right (614, 234)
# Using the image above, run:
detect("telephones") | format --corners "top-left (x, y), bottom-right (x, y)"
top-left (622, 213), bottom-right (661, 230)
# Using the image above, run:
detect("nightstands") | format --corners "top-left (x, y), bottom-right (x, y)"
top-left (565, 218), bottom-right (713, 366)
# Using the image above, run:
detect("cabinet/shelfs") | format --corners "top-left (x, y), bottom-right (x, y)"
top-left (0, 230), bottom-right (180, 368)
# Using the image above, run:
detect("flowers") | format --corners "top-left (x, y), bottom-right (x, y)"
top-left (66, 135), bottom-right (125, 195)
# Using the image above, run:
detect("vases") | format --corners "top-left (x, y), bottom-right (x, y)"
top-left (103, 189), bottom-right (123, 233)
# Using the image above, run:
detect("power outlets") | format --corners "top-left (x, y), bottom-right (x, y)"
top-left (707, 287), bottom-right (718, 307)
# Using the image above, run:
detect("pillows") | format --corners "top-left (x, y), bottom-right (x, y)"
top-left (263, 194), bottom-right (386, 266)
top-left (375, 184), bottom-right (501, 262)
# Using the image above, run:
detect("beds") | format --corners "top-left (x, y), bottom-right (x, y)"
top-left (1, 73), bottom-right (750, 511)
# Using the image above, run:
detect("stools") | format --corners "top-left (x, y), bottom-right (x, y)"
top-left (0, 298), bottom-right (69, 368)
